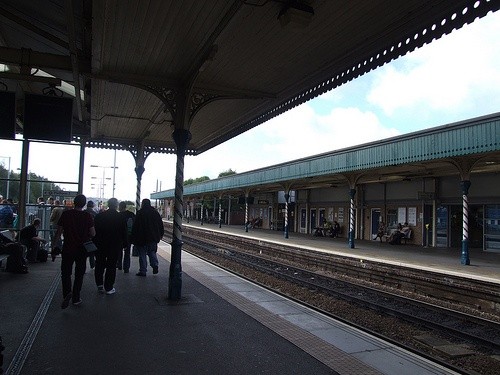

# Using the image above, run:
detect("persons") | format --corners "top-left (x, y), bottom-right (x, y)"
top-left (248, 217), bottom-right (262, 229)
top-left (82, 201), bottom-right (99, 274)
top-left (388, 222), bottom-right (409, 244)
top-left (51, 195), bottom-right (96, 310)
top-left (35, 196), bottom-right (68, 253)
top-left (373, 222), bottom-right (385, 243)
top-left (315, 218), bottom-right (327, 236)
top-left (94, 198), bottom-right (128, 295)
top-left (114, 202), bottom-right (137, 272)
top-left (15, 217), bottom-right (46, 259)
top-left (131, 199), bottom-right (164, 276)
top-left (330, 221), bottom-right (341, 239)
top-left (0, 195), bottom-right (28, 229)
top-left (0, 233), bottom-right (29, 274)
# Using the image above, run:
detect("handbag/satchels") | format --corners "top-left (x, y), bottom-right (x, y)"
top-left (81, 241), bottom-right (98, 254)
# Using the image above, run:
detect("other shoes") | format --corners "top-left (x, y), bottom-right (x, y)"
top-left (74, 298), bottom-right (83, 304)
top-left (17, 266), bottom-right (29, 273)
top-left (124, 269), bottom-right (129, 273)
top-left (136, 272), bottom-right (146, 276)
top-left (153, 266), bottom-right (158, 274)
top-left (62, 292), bottom-right (71, 309)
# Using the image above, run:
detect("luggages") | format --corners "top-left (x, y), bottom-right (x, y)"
top-left (40, 244), bottom-right (48, 262)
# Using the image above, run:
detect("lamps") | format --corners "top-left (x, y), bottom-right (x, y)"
top-left (277, 3), bottom-right (315, 33)
top-left (402, 178), bottom-right (411, 183)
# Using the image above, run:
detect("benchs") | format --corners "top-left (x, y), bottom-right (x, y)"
top-left (383, 229), bottom-right (411, 244)
top-left (0, 230), bottom-right (26, 271)
top-left (247, 220), bottom-right (263, 229)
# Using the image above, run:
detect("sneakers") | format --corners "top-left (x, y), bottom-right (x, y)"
top-left (98, 285), bottom-right (105, 292)
top-left (107, 288), bottom-right (116, 294)
